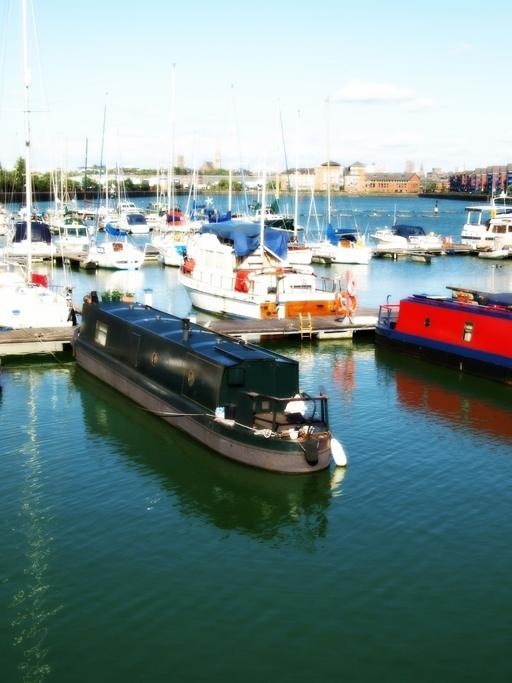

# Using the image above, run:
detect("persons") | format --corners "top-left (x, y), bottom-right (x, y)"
top-left (282, 391), bottom-right (308, 421)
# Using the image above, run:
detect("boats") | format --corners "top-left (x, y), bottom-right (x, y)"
top-left (370, 224), bottom-right (443, 250)
top-left (375, 284), bottom-right (512, 368)
top-left (71, 293), bottom-right (331, 474)
top-left (458, 187), bottom-right (512, 259)
